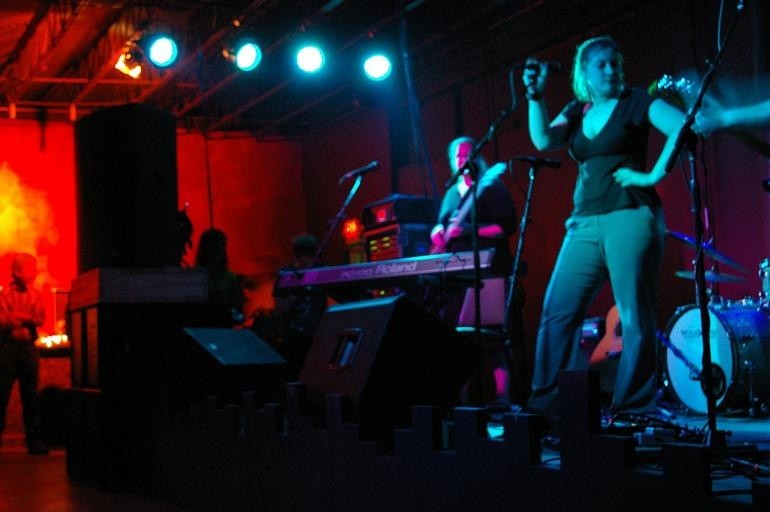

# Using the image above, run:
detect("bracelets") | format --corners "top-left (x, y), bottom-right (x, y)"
top-left (525, 92), bottom-right (546, 101)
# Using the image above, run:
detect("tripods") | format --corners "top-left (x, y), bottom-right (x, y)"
top-left (635, 0), bottom-right (770, 474)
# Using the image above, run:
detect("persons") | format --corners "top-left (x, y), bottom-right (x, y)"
top-left (1, 252), bottom-right (50, 457)
top-left (102, 214), bottom-right (327, 402)
top-left (680, 98), bottom-right (770, 133)
top-left (427, 136), bottom-right (518, 414)
top-left (523, 35), bottom-right (691, 414)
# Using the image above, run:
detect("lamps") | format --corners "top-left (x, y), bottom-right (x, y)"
top-left (141, 31), bottom-right (394, 84)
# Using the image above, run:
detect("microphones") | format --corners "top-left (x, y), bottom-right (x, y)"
top-left (516, 156), bottom-right (561, 168)
top-left (338, 160), bottom-right (380, 184)
top-left (524, 60), bottom-right (562, 73)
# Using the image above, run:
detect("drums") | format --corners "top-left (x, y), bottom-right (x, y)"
top-left (659, 300), bottom-right (769, 415)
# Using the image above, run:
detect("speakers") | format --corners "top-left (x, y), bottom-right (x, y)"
top-left (144, 328), bottom-right (292, 414)
top-left (296, 294), bottom-right (486, 452)
top-left (73, 102), bottom-right (182, 270)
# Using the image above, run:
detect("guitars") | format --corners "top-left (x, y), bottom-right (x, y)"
top-left (657, 74), bottom-right (770, 193)
top-left (591, 305), bottom-right (623, 363)
top-left (430, 162), bottom-right (508, 254)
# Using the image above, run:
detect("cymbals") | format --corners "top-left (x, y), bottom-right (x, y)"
top-left (674, 273), bottom-right (748, 284)
top-left (664, 229), bottom-right (751, 276)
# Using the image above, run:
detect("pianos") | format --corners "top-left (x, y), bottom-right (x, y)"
top-left (274, 247), bottom-right (515, 297)
